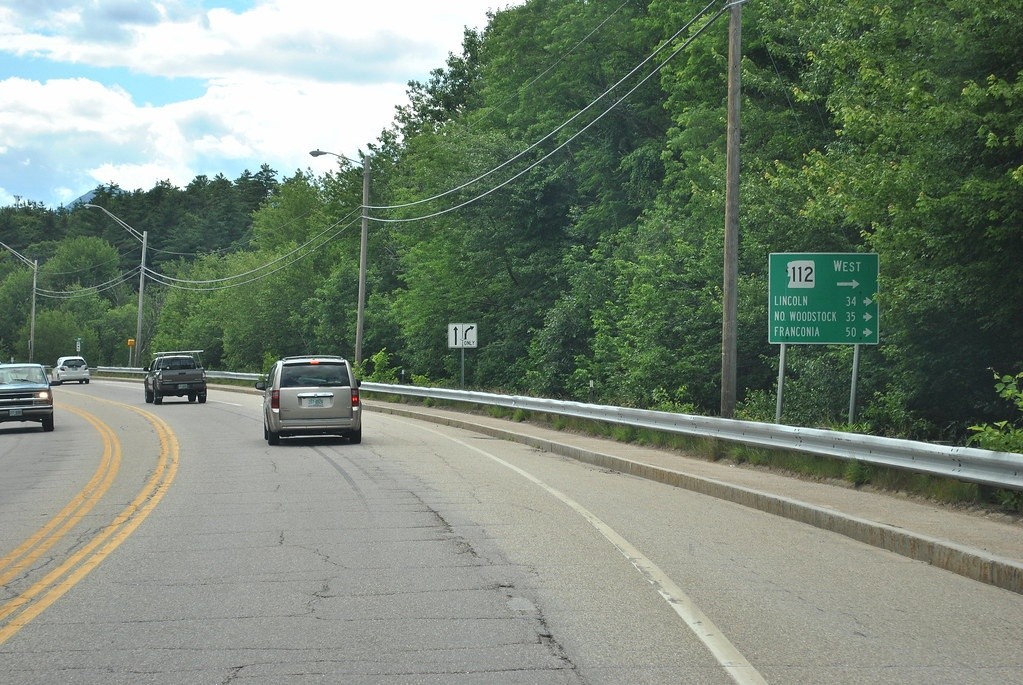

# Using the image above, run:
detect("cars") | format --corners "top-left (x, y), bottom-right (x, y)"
top-left (50, 355), bottom-right (90, 384)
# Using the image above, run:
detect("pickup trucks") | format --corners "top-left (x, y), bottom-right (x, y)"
top-left (143, 356), bottom-right (208, 405)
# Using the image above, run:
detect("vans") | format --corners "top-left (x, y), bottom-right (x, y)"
top-left (255, 354), bottom-right (361, 445)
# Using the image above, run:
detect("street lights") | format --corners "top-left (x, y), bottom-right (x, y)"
top-left (84, 202), bottom-right (149, 367)
top-left (310, 148), bottom-right (371, 369)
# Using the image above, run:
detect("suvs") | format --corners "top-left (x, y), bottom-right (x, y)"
top-left (0, 362), bottom-right (60, 432)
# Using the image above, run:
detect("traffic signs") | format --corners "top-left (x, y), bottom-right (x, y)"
top-left (768, 253), bottom-right (879, 346)
